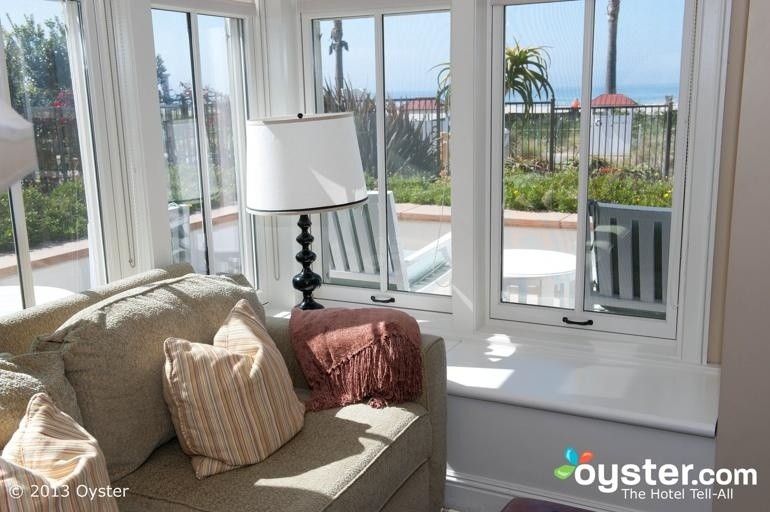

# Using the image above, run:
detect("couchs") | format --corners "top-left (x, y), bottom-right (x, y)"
top-left (0, 262), bottom-right (448, 512)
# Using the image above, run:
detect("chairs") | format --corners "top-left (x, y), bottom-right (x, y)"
top-left (588, 198), bottom-right (671, 319)
top-left (315, 189), bottom-right (456, 292)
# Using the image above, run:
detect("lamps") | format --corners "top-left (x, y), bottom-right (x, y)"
top-left (243, 110), bottom-right (368, 306)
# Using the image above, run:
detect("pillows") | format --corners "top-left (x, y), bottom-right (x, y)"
top-left (0, 388), bottom-right (122, 512)
top-left (159, 299), bottom-right (312, 481)
